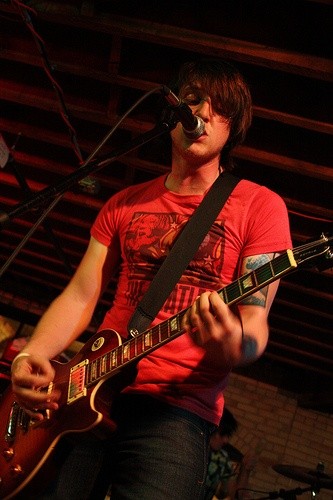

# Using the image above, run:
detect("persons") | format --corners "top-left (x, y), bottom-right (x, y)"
top-left (206, 408), bottom-right (246, 500)
top-left (11, 52), bottom-right (293, 500)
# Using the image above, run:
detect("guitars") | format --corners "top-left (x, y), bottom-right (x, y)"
top-left (0, 226), bottom-right (333, 500)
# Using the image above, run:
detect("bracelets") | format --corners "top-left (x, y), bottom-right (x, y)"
top-left (11, 353), bottom-right (31, 369)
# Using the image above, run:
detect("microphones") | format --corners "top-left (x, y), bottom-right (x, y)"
top-left (159, 85), bottom-right (205, 140)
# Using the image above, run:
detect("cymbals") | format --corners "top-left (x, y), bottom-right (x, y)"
top-left (272, 463), bottom-right (332, 490)
